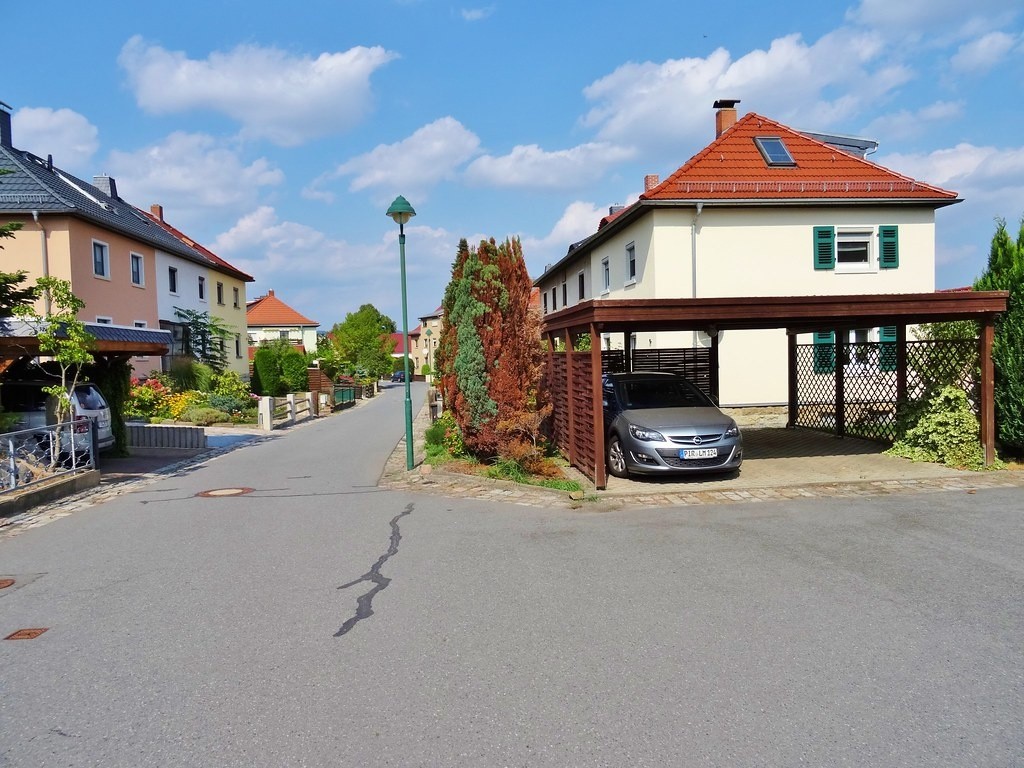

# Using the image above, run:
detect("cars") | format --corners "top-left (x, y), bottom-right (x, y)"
top-left (600, 371), bottom-right (744, 478)
top-left (389, 371), bottom-right (413, 382)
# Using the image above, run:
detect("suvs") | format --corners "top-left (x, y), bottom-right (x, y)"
top-left (0, 379), bottom-right (117, 456)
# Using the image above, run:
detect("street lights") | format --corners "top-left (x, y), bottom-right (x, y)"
top-left (387, 194), bottom-right (420, 470)
